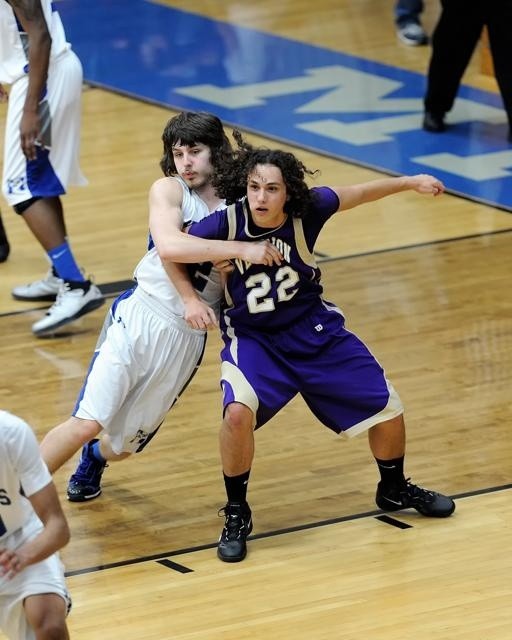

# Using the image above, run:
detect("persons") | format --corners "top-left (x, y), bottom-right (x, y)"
top-left (159, 128), bottom-right (457, 561)
top-left (423, 1), bottom-right (511, 143)
top-left (1, 411), bottom-right (73, 640)
top-left (1, 0), bottom-right (106, 336)
top-left (20, 112), bottom-right (285, 502)
top-left (393, 0), bottom-right (430, 46)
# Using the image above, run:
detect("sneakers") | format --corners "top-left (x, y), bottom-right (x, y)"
top-left (31, 278), bottom-right (109, 339)
top-left (11, 266), bottom-right (67, 302)
top-left (216, 501), bottom-right (254, 563)
top-left (65, 437), bottom-right (109, 503)
top-left (375, 479), bottom-right (456, 519)
top-left (424, 108), bottom-right (446, 133)
top-left (394, 22), bottom-right (430, 48)
